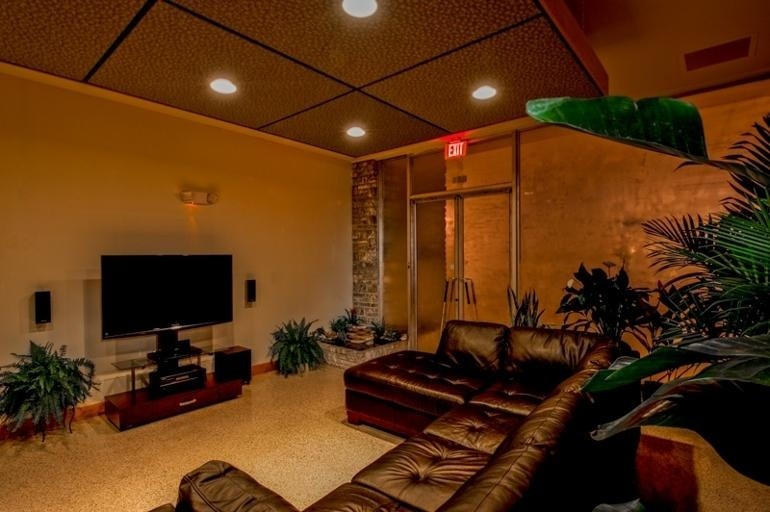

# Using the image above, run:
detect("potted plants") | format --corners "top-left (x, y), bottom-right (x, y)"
top-left (267, 319), bottom-right (327, 379)
top-left (0, 340), bottom-right (101, 443)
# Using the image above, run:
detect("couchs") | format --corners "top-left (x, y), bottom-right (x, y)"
top-left (152, 318), bottom-right (640, 510)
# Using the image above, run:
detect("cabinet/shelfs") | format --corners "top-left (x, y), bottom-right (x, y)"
top-left (104, 345), bottom-right (252, 432)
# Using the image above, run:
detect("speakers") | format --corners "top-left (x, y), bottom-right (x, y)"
top-left (34, 289), bottom-right (52, 325)
top-left (215, 345), bottom-right (252, 384)
top-left (246, 279), bottom-right (256, 302)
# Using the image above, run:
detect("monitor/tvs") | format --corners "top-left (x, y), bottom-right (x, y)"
top-left (100, 251), bottom-right (234, 361)
